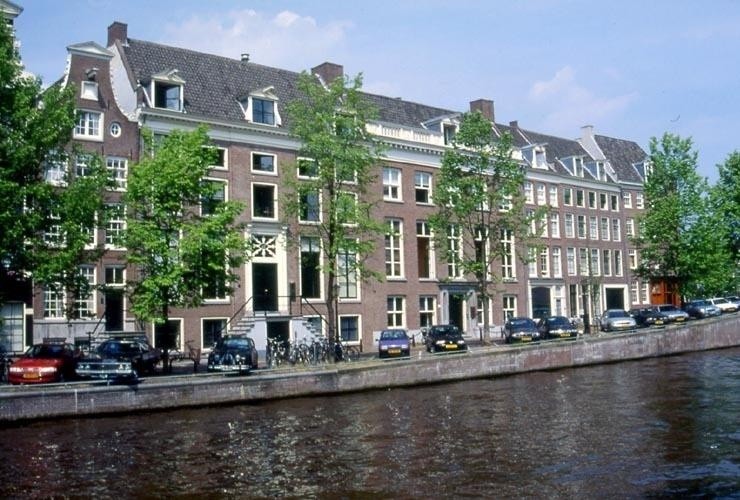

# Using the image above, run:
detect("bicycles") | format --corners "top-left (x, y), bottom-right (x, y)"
top-left (266, 334), bottom-right (360, 370)
top-left (168, 339), bottom-right (200, 361)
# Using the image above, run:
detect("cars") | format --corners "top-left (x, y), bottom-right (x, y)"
top-left (376, 328), bottom-right (413, 359)
top-left (425, 324), bottom-right (467, 354)
top-left (599, 295), bottom-right (740, 332)
top-left (7, 337), bottom-right (162, 383)
top-left (207, 336), bottom-right (258, 371)
top-left (502, 316), bottom-right (579, 344)
